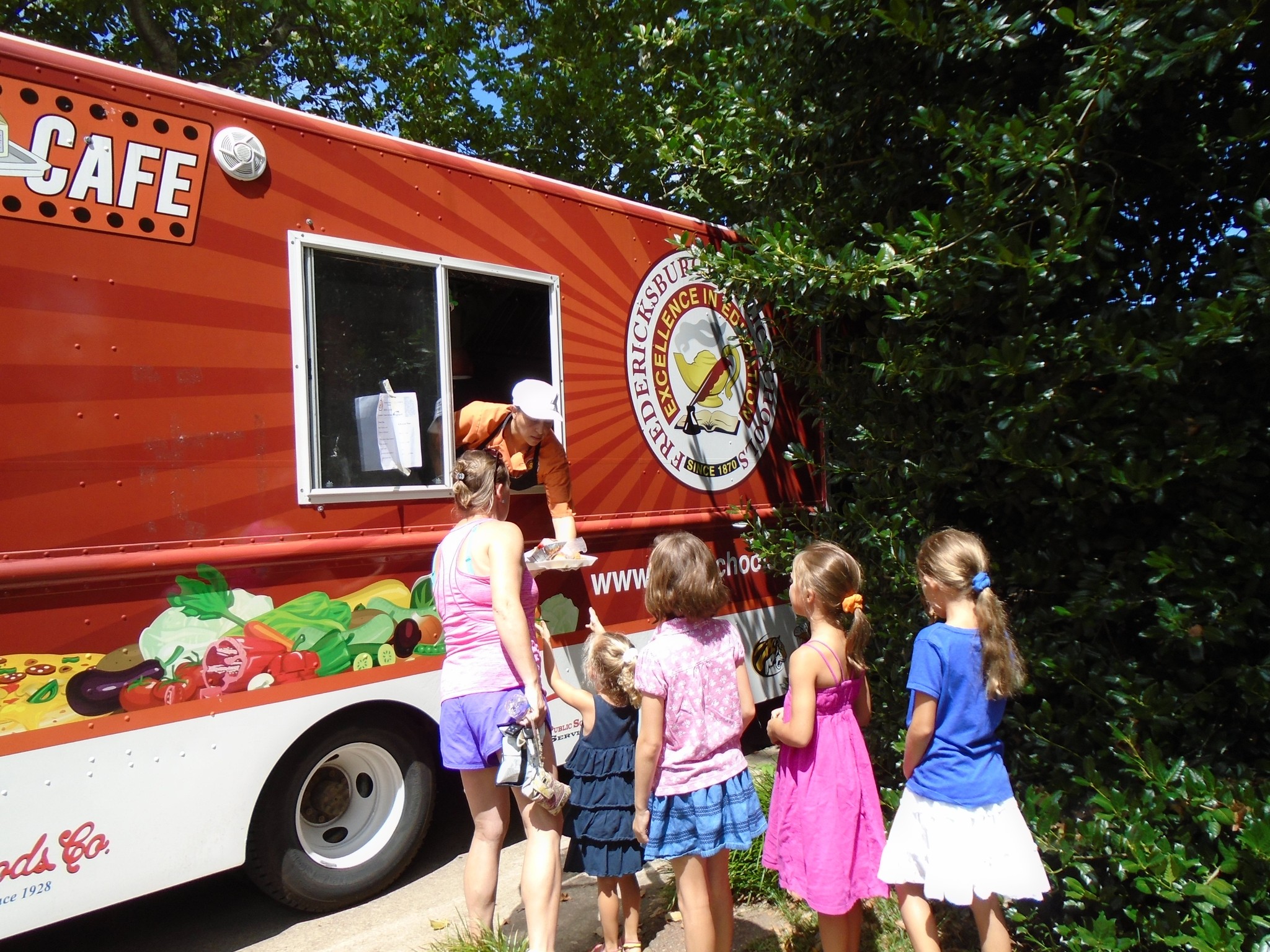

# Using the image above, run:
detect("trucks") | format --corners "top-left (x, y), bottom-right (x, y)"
top-left (0, 32), bottom-right (828, 952)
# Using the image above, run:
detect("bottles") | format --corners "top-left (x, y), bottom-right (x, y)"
top-left (505, 694), bottom-right (532, 721)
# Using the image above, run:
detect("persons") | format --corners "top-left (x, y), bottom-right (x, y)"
top-left (534, 608), bottom-right (646, 952)
top-left (762, 544), bottom-right (891, 952)
top-left (435, 380), bottom-right (577, 571)
top-left (633, 535), bottom-right (768, 952)
top-left (432, 452), bottom-right (565, 952)
top-left (877, 530), bottom-right (1050, 952)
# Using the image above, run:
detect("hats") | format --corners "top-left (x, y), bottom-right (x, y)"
top-left (512, 378), bottom-right (563, 421)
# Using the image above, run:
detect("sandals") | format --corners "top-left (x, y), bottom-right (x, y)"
top-left (618, 937), bottom-right (642, 952)
top-left (591, 943), bottom-right (622, 952)
